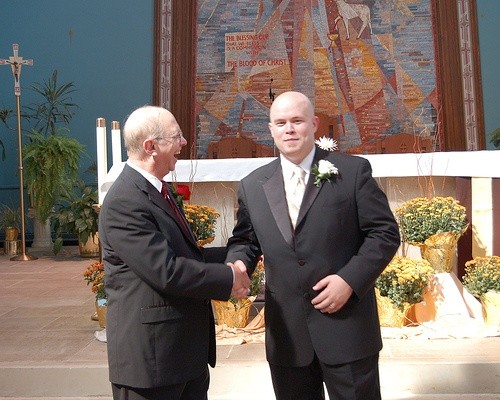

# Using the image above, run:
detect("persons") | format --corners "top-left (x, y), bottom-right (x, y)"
top-left (97, 105), bottom-right (249, 400)
top-left (224, 91), bottom-right (401, 400)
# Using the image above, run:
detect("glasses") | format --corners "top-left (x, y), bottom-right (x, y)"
top-left (154, 130), bottom-right (182, 142)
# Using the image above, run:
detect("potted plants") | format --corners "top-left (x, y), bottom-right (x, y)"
top-left (0, 71), bottom-right (90, 251)
top-left (0, 203), bottom-right (27, 241)
top-left (51, 187), bottom-right (100, 258)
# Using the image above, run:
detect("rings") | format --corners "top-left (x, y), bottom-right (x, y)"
top-left (329, 305), bottom-right (334, 308)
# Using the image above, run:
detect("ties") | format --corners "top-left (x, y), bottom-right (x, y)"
top-left (161, 182), bottom-right (189, 235)
top-left (287, 167), bottom-right (306, 229)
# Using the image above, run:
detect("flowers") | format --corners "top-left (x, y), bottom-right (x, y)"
top-left (228, 255), bottom-right (264, 309)
top-left (462, 256), bottom-right (500, 298)
top-left (83, 262), bottom-right (108, 300)
top-left (375, 256), bottom-right (434, 311)
top-left (312, 159), bottom-right (339, 187)
top-left (172, 184), bottom-right (220, 245)
top-left (395, 196), bottom-right (469, 246)
top-left (316, 137), bottom-right (337, 152)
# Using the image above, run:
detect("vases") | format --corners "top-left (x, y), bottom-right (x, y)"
top-left (213, 296), bottom-right (256, 329)
top-left (420, 232), bottom-right (456, 272)
top-left (375, 287), bottom-right (417, 327)
top-left (95, 299), bottom-right (108, 328)
top-left (480, 290), bottom-right (500, 326)
